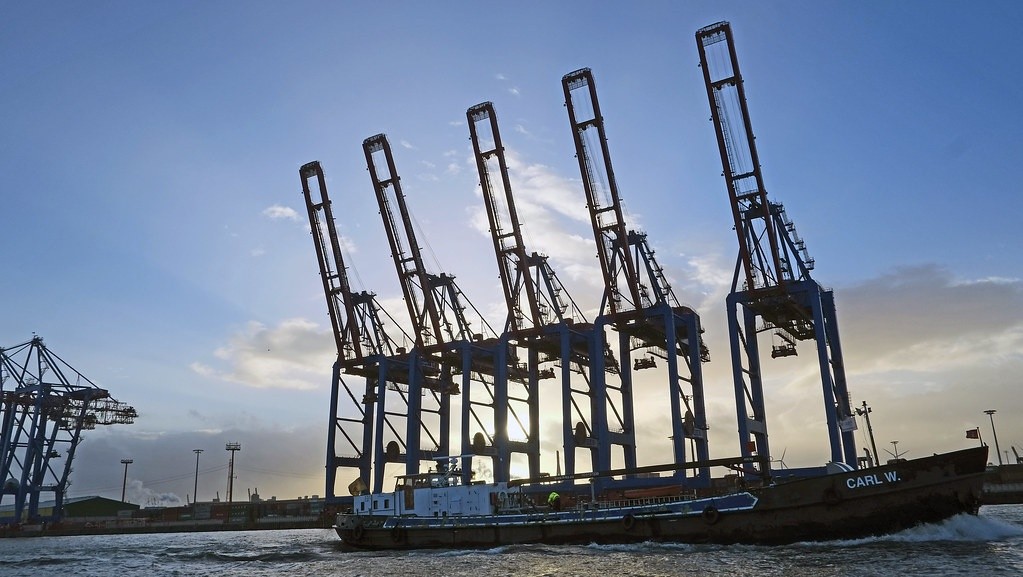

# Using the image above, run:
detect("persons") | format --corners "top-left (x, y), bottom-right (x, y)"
top-left (548, 491), bottom-right (561, 512)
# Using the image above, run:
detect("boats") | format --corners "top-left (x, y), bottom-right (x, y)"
top-left (332, 444), bottom-right (992, 550)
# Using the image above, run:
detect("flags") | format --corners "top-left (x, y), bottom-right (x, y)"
top-left (966, 429), bottom-right (978, 439)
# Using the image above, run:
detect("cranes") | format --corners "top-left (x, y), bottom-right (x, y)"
top-left (0, 332), bottom-right (140, 527)
top-left (299, 99), bottom-right (624, 502)
top-left (558, 65), bottom-right (710, 491)
top-left (694, 18), bottom-right (858, 490)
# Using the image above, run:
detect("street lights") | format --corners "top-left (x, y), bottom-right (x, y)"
top-left (854, 400), bottom-right (879, 466)
top-left (225, 440), bottom-right (242, 503)
top-left (121, 457), bottom-right (133, 502)
top-left (191, 449), bottom-right (203, 520)
top-left (982, 409), bottom-right (1002, 466)
